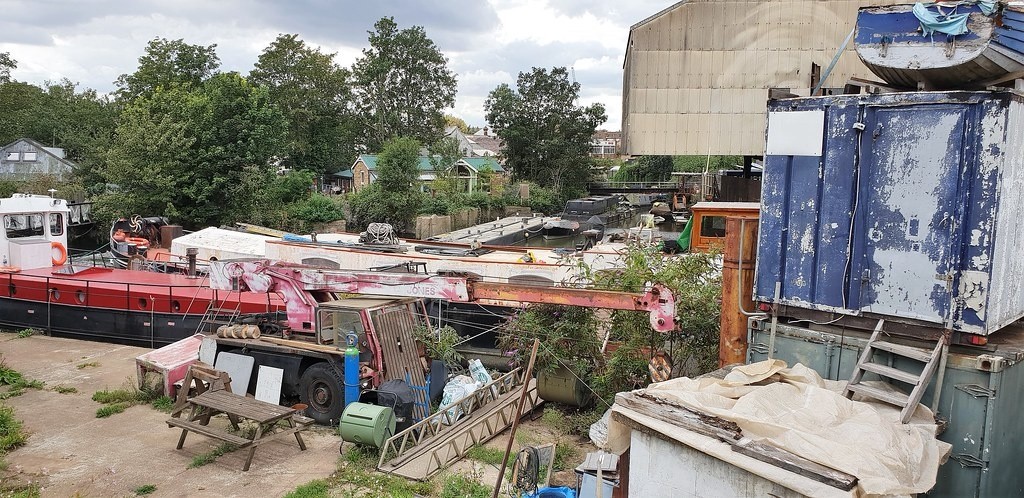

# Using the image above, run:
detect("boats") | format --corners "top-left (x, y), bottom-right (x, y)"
top-left (541, 218), bottom-right (580, 241)
top-left (1, 193), bottom-right (292, 351)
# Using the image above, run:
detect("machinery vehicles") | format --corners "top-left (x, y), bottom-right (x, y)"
top-left (198, 258), bottom-right (685, 429)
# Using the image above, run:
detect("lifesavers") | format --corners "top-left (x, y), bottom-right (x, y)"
top-left (0, 266), bottom-right (19, 274)
top-left (51, 241), bottom-right (66, 266)
top-left (126, 237), bottom-right (149, 246)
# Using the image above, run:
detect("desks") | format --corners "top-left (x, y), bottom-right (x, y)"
top-left (135, 333), bottom-right (210, 398)
top-left (177, 389), bottom-right (306, 472)
top-left (293, 413), bottom-right (316, 427)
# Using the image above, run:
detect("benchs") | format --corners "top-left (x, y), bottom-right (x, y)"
top-left (166, 418), bottom-right (253, 448)
